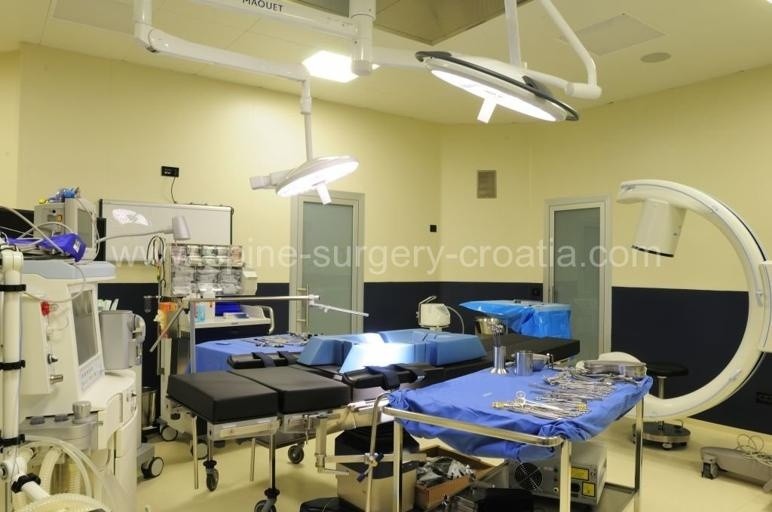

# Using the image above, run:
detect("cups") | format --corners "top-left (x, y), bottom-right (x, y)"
top-left (515, 348), bottom-right (533, 377)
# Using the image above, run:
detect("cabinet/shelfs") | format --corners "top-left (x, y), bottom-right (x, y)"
top-left (382, 359), bottom-right (653, 511)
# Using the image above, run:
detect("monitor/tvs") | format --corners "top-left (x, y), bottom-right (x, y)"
top-left (33, 198), bottom-right (98, 261)
top-left (49, 279), bottom-right (105, 398)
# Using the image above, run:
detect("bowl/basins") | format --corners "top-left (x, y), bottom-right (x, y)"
top-left (512, 352), bottom-right (547, 371)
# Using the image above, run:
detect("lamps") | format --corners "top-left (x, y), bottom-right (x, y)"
top-left (92, 216), bottom-right (192, 247)
top-left (414, 52), bottom-right (578, 127)
top-left (248, 156), bottom-right (359, 206)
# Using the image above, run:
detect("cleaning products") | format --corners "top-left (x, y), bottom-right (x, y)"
top-left (202, 286), bottom-right (215, 318)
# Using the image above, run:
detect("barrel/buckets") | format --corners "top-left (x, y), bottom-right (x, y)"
top-left (142, 387), bottom-right (160, 429)
